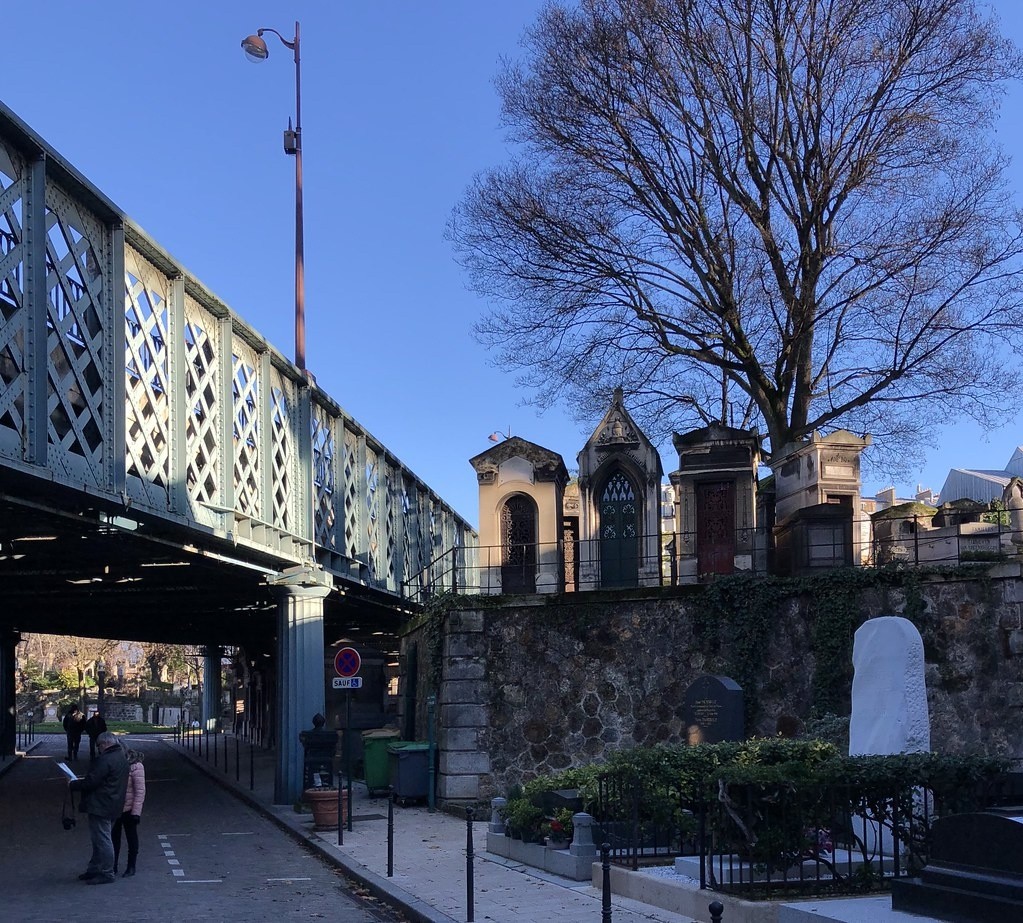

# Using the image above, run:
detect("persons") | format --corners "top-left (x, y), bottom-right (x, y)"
top-left (65, 733), bottom-right (131, 884)
top-left (112, 737), bottom-right (145, 877)
top-left (87, 711), bottom-right (107, 761)
top-left (63, 704), bottom-right (86, 761)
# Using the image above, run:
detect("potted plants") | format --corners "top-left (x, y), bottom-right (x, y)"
top-left (493, 798), bottom-right (574, 850)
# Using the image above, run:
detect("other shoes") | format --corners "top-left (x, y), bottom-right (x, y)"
top-left (78, 872), bottom-right (116, 885)
top-left (123, 865), bottom-right (135, 877)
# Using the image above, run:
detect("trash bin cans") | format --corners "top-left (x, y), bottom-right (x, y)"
top-left (360, 729), bottom-right (402, 797)
top-left (386, 742), bottom-right (436, 807)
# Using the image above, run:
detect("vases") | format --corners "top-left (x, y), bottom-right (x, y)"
top-left (304, 785), bottom-right (351, 832)
